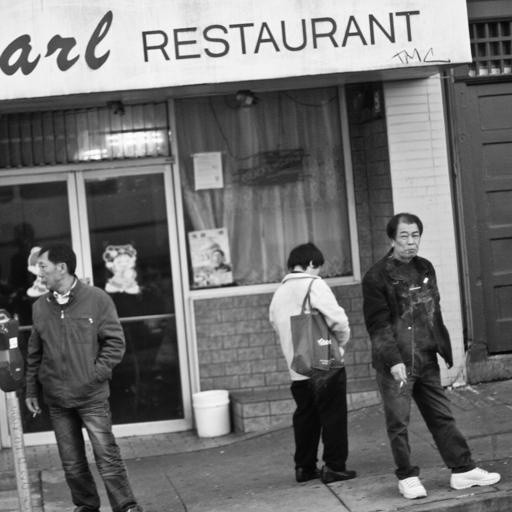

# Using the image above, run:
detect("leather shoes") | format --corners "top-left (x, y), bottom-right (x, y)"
top-left (296, 466), bottom-right (321, 481)
top-left (320, 465), bottom-right (355, 483)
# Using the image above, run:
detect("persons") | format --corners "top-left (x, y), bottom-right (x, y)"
top-left (362, 213), bottom-right (501, 500)
top-left (25, 241), bottom-right (145, 511)
top-left (9, 222), bottom-right (36, 287)
top-left (211, 249), bottom-right (230, 272)
top-left (268, 242), bottom-right (359, 483)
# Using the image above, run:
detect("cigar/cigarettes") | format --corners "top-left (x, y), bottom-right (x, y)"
top-left (399, 379), bottom-right (404, 390)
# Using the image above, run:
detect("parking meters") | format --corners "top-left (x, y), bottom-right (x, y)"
top-left (0, 309), bottom-right (36, 512)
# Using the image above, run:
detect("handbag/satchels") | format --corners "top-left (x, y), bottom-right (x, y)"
top-left (290, 313), bottom-right (345, 380)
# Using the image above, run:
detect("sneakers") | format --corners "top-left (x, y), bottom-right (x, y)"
top-left (450, 467), bottom-right (501, 490)
top-left (398, 476), bottom-right (427, 499)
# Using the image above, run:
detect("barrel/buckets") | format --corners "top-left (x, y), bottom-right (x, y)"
top-left (192, 390), bottom-right (230, 439)
